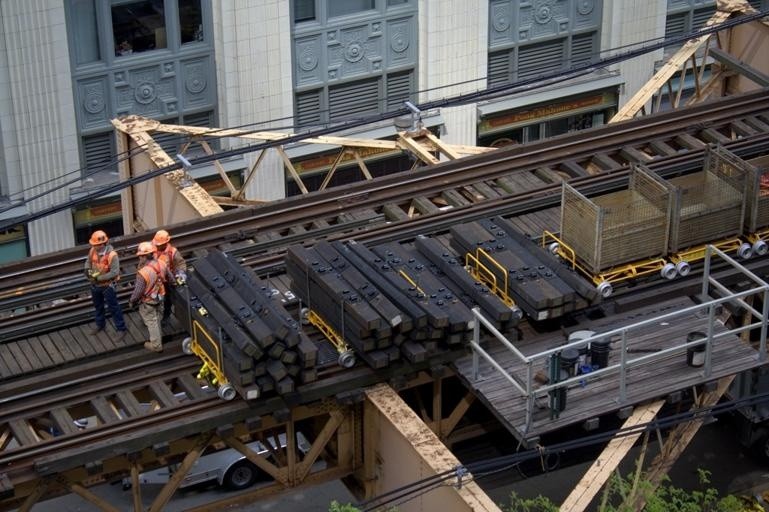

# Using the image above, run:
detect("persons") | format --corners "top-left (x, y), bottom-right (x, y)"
top-left (83, 230), bottom-right (128, 343)
top-left (129, 242), bottom-right (177, 353)
top-left (143, 229), bottom-right (187, 326)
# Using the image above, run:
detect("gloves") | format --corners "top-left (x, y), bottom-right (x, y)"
top-left (178, 270), bottom-right (187, 282)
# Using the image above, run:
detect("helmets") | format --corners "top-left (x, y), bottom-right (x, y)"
top-left (136, 241), bottom-right (158, 256)
top-left (89, 230), bottom-right (109, 246)
top-left (152, 229), bottom-right (171, 247)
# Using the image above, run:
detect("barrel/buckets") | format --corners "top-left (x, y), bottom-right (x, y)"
top-left (570, 338), bottom-right (588, 371)
top-left (591, 333), bottom-right (609, 368)
top-left (687, 330), bottom-right (707, 366)
top-left (548, 389), bottom-right (567, 410)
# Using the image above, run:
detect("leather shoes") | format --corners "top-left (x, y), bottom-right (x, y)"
top-left (144, 342), bottom-right (162, 352)
top-left (90, 326), bottom-right (101, 335)
top-left (115, 332), bottom-right (127, 343)
